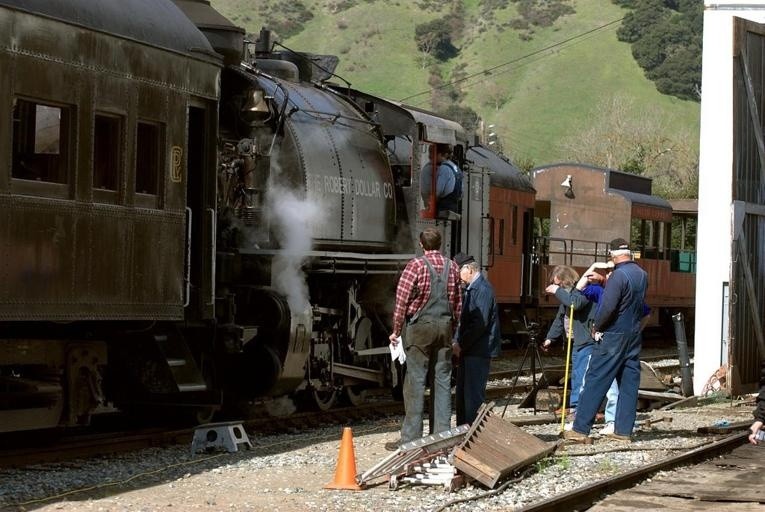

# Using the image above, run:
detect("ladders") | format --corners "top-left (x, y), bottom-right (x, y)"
top-left (353, 421), bottom-right (472, 485)
top-left (449, 399), bottom-right (566, 491)
top-left (387, 446), bottom-right (466, 492)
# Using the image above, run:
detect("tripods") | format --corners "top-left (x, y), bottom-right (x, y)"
top-left (502, 337), bottom-right (559, 424)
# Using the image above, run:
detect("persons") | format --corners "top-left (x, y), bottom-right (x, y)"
top-left (748, 360), bottom-right (765, 445)
top-left (436, 145), bottom-right (464, 213)
top-left (451, 253), bottom-right (502, 428)
top-left (564, 257), bottom-right (651, 435)
top-left (541, 265), bottom-right (598, 414)
top-left (559, 238), bottom-right (649, 444)
top-left (385, 227), bottom-right (463, 452)
top-left (420, 145), bottom-right (433, 210)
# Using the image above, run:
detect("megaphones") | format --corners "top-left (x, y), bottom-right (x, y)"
top-left (560, 175), bottom-right (571, 188)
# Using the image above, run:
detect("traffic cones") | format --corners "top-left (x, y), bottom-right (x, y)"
top-left (323, 427), bottom-right (368, 491)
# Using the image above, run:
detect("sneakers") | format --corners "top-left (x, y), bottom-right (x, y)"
top-left (563, 429), bottom-right (588, 442)
top-left (599, 422), bottom-right (615, 434)
top-left (385, 441), bottom-right (402, 450)
top-left (565, 421), bottom-right (574, 430)
top-left (605, 432), bottom-right (632, 443)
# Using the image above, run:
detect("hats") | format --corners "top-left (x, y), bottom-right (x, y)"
top-left (611, 238), bottom-right (629, 251)
top-left (453, 251), bottom-right (475, 267)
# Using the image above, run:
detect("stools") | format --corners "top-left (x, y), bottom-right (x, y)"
top-left (190, 419), bottom-right (253, 457)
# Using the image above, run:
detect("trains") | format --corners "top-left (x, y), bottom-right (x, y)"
top-left (0, 1), bottom-right (698, 437)
